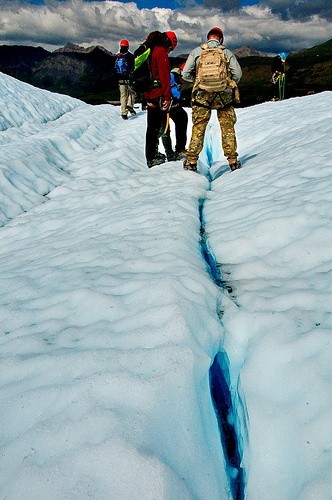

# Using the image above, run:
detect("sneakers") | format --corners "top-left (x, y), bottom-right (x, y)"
top-left (227, 157), bottom-right (242, 171)
top-left (183, 157), bottom-right (197, 172)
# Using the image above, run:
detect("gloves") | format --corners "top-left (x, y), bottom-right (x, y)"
top-left (163, 99), bottom-right (170, 108)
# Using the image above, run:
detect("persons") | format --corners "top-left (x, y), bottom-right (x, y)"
top-left (271, 55), bottom-right (285, 101)
top-left (114, 31), bottom-right (188, 168)
top-left (181, 27), bottom-right (243, 172)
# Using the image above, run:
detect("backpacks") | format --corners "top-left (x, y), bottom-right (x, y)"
top-left (128, 44), bottom-right (160, 93)
top-left (194, 44), bottom-right (230, 93)
top-left (114, 51), bottom-right (129, 74)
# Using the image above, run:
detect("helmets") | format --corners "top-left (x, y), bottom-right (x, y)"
top-left (120, 40), bottom-right (129, 46)
top-left (164, 32), bottom-right (177, 51)
top-left (207, 26), bottom-right (223, 44)
top-left (179, 64), bottom-right (185, 71)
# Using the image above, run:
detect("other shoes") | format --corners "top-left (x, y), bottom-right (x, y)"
top-left (126, 106), bottom-right (136, 115)
top-left (176, 149), bottom-right (187, 160)
top-left (122, 115), bottom-right (128, 120)
top-left (166, 151), bottom-right (175, 161)
top-left (147, 155), bottom-right (165, 168)
